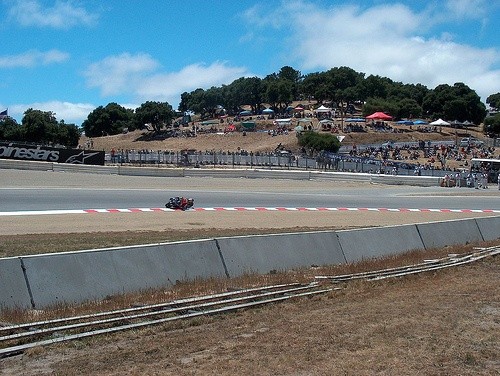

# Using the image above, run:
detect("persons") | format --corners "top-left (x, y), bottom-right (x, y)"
top-left (180, 197), bottom-right (187, 211)
top-left (78, 97), bottom-right (500, 191)
top-left (174, 196), bottom-right (181, 206)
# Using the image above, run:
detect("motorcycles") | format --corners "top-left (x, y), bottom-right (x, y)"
top-left (165, 198), bottom-right (194, 209)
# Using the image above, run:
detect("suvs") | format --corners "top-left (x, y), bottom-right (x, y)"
top-left (461, 138), bottom-right (484, 147)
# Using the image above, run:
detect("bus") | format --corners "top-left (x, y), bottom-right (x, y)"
top-left (471, 158), bottom-right (500, 175)
